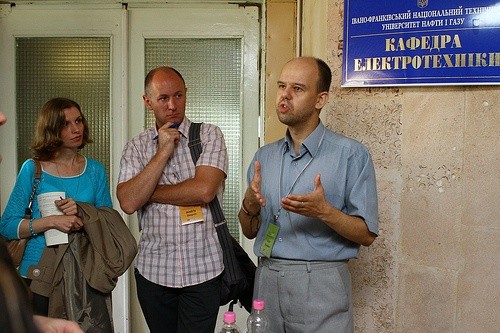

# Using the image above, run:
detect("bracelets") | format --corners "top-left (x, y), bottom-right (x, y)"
top-left (241, 199), bottom-right (261, 216)
top-left (29, 218), bottom-right (38, 238)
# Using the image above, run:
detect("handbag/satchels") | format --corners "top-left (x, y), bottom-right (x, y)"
top-left (4, 157), bottom-right (42, 270)
top-left (221, 234), bottom-right (257, 314)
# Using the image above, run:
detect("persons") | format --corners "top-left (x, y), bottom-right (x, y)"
top-left (117, 66), bottom-right (228, 333)
top-left (0, 97), bottom-right (106, 333)
top-left (238, 54), bottom-right (378, 333)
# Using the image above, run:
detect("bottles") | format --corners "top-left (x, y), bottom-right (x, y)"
top-left (219, 311), bottom-right (242, 333)
top-left (246, 300), bottom-right (268, 333)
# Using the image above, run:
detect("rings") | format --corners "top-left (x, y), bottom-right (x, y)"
top-left (301, 201), bottom-right (304, 208)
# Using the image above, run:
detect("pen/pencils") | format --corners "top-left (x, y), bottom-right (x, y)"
top-left (60, 196), bottom-right (63, 200)
top-left (153, 121), bottom-right (179, 141)
